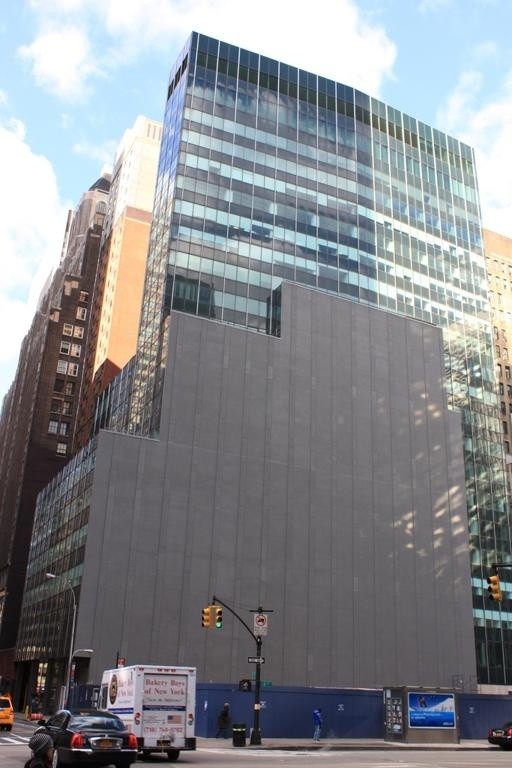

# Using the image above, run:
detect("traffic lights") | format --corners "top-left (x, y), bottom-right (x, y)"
top-left (486, 574), bottom-right (502, 602)
top-left (241, 680), bottom-right (251, 690)
top-left (202, 607), bottom-right (211, 628)
top-left (215, 608), bottom-right (223, 628)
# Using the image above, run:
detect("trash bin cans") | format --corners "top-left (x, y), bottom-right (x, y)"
top-left (232, 723), bottom-right (247, 747)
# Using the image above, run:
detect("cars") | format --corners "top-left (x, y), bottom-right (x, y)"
top-left (486, 721), bottom-right (511, 751)
top-left (33, 707), bottom-right (144, 768)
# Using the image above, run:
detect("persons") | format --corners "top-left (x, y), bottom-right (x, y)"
top-left (214, 702), bottom-right (232, 739)
top-left (311, 707), bottom-right (324, 741)
top-left (24, 732), bottom-right (55, 768)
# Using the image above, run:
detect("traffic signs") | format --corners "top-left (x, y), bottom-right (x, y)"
top-left (260, 680), bottom-right (272, 686)
top-left (247, 656), bottom-right (266, 665)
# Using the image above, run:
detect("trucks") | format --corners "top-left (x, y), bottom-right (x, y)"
top-left (98, 664), bottom-right (198, 760)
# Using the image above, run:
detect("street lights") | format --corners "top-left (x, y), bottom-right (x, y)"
top-left (46, 572), bottom-right (94, 704)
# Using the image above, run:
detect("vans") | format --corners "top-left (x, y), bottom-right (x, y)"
top-left (0, 693), bottom-right (17, 732)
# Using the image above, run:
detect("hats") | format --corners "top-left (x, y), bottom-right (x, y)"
top-left (29, 734), bottom-right (51, 755)
